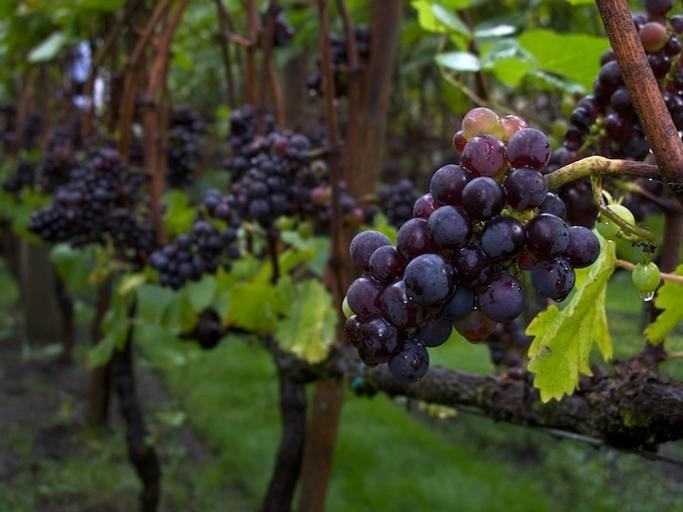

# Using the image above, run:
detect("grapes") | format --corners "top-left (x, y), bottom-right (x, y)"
top-left (1, 8), bottom-right (683, 383)
